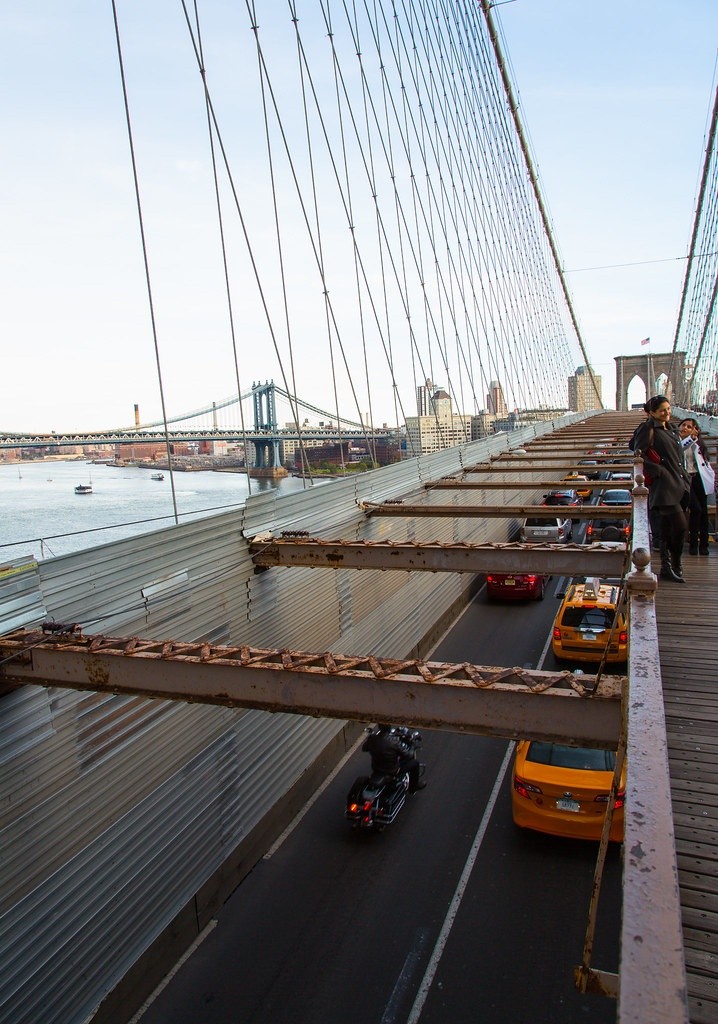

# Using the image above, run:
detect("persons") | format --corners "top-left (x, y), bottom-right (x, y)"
top-left (679, 418), bottom-right (716, 556)
top-left (361, 724), bottom-right (426, 793)
top-left (629, 395), bottom-right (688, 582)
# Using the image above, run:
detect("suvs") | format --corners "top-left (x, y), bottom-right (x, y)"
top-left (511, 740), bottom-right (626, 842)
top-left (552, 577), bottom-right (631, 662)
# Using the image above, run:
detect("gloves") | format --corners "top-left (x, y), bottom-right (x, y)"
top-left (412, 741), bottom-right (422, 751)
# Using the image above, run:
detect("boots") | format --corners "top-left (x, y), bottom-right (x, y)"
top-left (670, 531), bottom-right (682, 577)
top-left (658, 538), bottom-right (684, 582)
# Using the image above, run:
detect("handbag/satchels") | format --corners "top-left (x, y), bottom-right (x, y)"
top-left (639, 422), bottom-right (661, 486)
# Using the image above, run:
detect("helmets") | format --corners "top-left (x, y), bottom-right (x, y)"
top-left (379, 723), bottom-right (391, 732)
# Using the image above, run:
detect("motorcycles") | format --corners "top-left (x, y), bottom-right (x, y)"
top-left (345, 725), bottom-right (425, 835)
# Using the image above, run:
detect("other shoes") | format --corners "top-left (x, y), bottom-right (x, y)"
top-left (689, 541), bottom-right (698, 555)
top-left (409, 782), bottom-right (427, 793)
top-left (699, 545), bottom-right (709, 556)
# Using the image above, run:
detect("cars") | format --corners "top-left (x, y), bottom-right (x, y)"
top-left (520, 438), bottom-right (634, 545)
top-left (486, 574), bottom-right (553, 601)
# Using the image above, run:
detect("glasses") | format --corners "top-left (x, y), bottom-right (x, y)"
top-left (651, 394), bottom-right (663, 403)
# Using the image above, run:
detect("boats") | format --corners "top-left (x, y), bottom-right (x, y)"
top-left (151, 472), bottom-right (164, 479)
top-left (75, 485), bottom-right (92, 494)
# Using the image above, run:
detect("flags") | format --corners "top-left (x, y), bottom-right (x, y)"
top-left (641, 338), bottom-right (651, 345)
